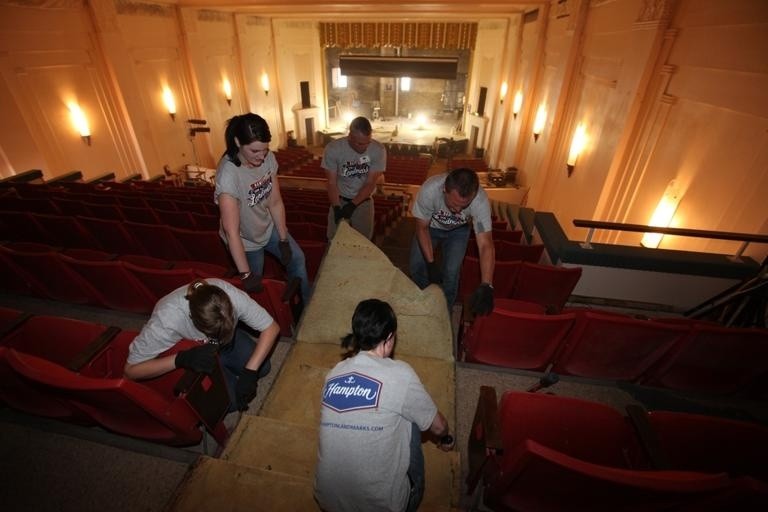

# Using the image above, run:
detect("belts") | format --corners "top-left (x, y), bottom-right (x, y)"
top-left (340, 196), bottom-right (352, 201)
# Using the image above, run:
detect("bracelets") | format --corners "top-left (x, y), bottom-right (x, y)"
top-left (240, 270), bottom-right (251, 280)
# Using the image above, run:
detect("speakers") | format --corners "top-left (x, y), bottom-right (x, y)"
top-left (300, 81), bottom-right (310, 108)
top-left (477, 87), bottom-right (487, 116)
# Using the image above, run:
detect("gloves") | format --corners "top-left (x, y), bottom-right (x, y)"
top-left (334, 206), bottom-right (343, 222)
top-left (427, 264), bottom-right (440, 283)
top-left (240, 272), bottom-right (257, 291)
top-left (343, 201), bottom-right (356, 219)
top-left (279, 241), bottom-right (290, 265)
top-left (234, 367), bottom-right (257, 403)
top-left (472, 286), bottom-right (494, 314)
top-left (175, 344), bottom-right (217, 373)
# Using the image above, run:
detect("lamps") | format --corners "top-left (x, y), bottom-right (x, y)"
top-left (500, 100), bottom-right (504, 104)
top-left (534, 133), bottom-right (539, 142)
top-left (227, 99), bottom-right (231, 105)
top-left (170, 113), bottom-right (176, 120)
top-left (513, 112), bottom-right (518, 120)
top-left (81, 133), bottom-right (91, 146)
top-left (265, 90), bottom-right (268, 96)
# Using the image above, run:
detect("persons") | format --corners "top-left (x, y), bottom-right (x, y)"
top-left (409, 167), bottom-right (498, 316)
top-left (320, 117), bottom-right (387, 244)
top-left (312, 298), bottom-right (456, 512)
top-left (123, 277), bottom-right (281, 414)
top-left (212, 112), bottom-right (311, 325)
